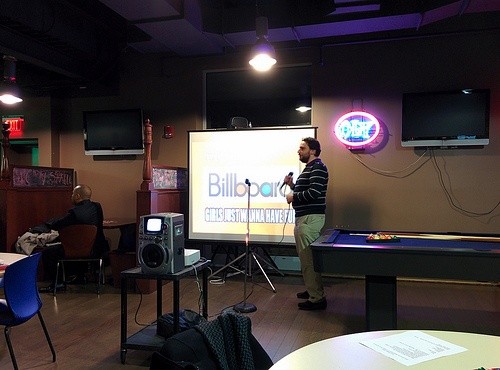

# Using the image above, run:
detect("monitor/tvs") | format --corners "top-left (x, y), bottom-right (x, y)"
top-left (82, 108), bottom-right (145, 156)
top-left (401, 88), bottom-right (492, 147)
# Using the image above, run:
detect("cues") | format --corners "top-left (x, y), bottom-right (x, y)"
top-left (349, 235), bottom-right (500, 241)
top-left (377, 232), bottom-right (445, 236)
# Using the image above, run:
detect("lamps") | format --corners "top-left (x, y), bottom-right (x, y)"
top-left (0, 55), bottom-right (23, 105)
top-left (246, 15), bottom-right (279, 71)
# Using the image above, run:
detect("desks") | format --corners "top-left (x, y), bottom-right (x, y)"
top-left (267, 329), bottom-right (500, 370)
top-left (308, 225), bottom-right (500, 332)
top-left (119, 259), bottom-right (212, 366)
top-left (101, 217), bottom-right (136, 254)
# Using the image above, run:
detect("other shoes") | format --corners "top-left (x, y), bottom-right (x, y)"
top-left (297, 300), bottom-right (327, 309)
top-left (297, 290), bottom-right (310, 298)
top-left (39, 281), bottom-right (65, 292)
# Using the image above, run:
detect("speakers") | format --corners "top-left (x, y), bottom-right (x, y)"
top-left (138, 213), bottom-right (185, 275)
top-left (226, 117), bottom-right (248, 128)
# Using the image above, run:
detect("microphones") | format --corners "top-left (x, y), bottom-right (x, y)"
top-left (280, 172), bottom-right (293, 188)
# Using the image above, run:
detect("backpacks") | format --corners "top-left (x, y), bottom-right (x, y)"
top-left (157, 309), bottom-right (206, 336)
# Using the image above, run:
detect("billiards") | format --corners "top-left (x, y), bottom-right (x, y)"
top-left (368, 232), bottom-right (397, 240)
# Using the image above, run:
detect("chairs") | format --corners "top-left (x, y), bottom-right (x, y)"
top-left (52, 222), bottom-right (106, 296)
top-left (0, 252), bottom-right (57, 370)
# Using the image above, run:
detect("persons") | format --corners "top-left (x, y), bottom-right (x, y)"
top-left (284, 137), bottom-right (329, 311)
top-left (38, 184), bottom-right (110, 294)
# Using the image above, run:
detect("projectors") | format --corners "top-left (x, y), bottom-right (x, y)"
top-left (184, 249), bottom-right (201, 266)
